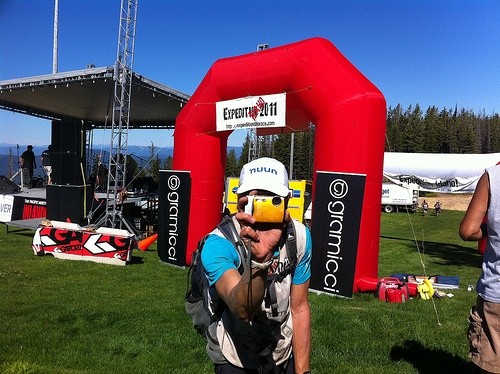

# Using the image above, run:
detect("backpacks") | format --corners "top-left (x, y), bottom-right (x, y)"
top-left (184, 215), bottom-right (298, 340)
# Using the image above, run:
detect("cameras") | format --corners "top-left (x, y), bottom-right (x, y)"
top-left (244, 195), bottom-right (285, 223)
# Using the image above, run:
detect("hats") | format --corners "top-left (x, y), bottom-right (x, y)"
top-left (27, 145), bottom-right (33, 148)
top-left (236, 157), bottom-right (289, 197)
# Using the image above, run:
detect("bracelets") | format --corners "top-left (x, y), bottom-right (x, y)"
top-left (249, 260), bottom-right (273, 269)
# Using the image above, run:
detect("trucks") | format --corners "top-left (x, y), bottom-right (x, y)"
top-left (380, 172), bottom-right (419, 213)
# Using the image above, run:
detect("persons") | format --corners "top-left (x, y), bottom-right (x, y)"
top-left (421, 200), bottom-right (428, 214)
top-left (40, 145), bottom-right (53, 185)
top-left (459, 161), bottom-right (500, 374)
top-left (185, 156), bottom-right (311, 374)
top-left (434, 200), bottom-right (441, 214)
top-left (20, 145), bottom-right (36, 187)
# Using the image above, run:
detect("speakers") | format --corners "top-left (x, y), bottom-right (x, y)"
top-left (46, 119), bottom-right (94, 227)
top-left (0, 175), bottom-right (21, 195)
